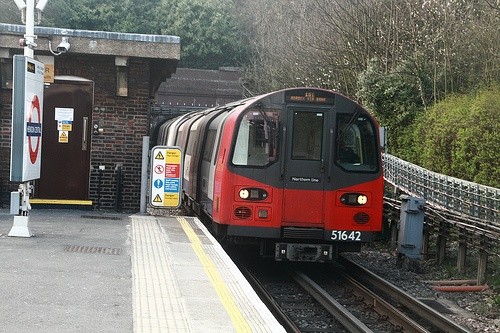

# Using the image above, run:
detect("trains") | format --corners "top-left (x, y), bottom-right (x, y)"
top-left (159, 86), bottom-right (389, 276)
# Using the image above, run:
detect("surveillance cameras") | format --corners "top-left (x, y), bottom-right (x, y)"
top-left (56, 37), bottom-right (71, 53)
